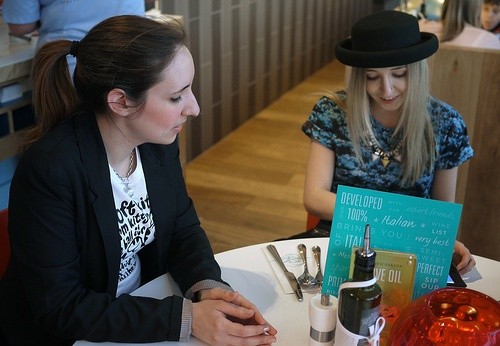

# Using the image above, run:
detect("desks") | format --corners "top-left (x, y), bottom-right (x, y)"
top-left (0, 15), bottom-right (188, 182)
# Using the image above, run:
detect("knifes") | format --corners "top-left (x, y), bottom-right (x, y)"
top-left (267, 245), bottom-right (303, 302)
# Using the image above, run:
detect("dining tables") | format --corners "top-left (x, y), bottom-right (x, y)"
top-left (72, 237), bottom-right (500, 346)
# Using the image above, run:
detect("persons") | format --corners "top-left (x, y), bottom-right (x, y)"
top-left (481, 0), bottom-right (500, 32)
top-left (0, 15), bottom-right (277, 346)
top-left (272, 10), bottom-right (477, 275)
top-left (4, 0), bottom-right (145, 87)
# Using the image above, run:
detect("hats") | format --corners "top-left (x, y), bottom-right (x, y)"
top-left (333, 9), bottom-right (438, 69)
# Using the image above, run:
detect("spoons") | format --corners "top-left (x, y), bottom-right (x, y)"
top-left (297, 244), bottom-right (317, 286)
top-left (312, 246), bottom-right (324, 287)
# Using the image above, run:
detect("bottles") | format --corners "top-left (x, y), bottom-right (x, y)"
top-left (308, 293), bottom-right (338, 346)
top-left (334, 224), bottom-right (381, 346)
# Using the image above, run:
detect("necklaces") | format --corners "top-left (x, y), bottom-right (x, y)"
top-left (370, 134), bottom-right (405, 166)
top-left (113, 149), bottom-right (133, 197)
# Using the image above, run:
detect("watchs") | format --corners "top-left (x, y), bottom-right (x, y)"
top-left (420, 0), bottom-right (500, 50)
